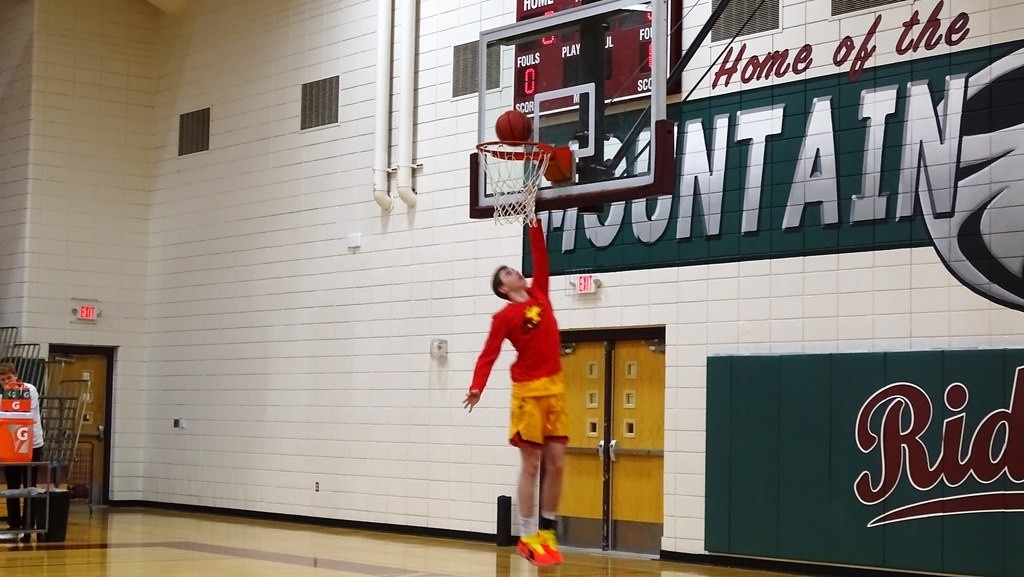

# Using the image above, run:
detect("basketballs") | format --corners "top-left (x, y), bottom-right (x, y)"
top-left (495, 110), bottom-right (532, 146)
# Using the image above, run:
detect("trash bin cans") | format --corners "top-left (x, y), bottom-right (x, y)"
top-left (36, 487), bottom-right (71, 542)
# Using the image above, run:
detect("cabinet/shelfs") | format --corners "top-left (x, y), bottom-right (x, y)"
top-left (0, 462), bottom-right (52, 547)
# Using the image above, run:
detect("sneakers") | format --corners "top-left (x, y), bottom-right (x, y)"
top-left (19, 532), bottom-right (31, 543)
top-left (0, 527), bottom-right (17, 539)
top-left (516, 536), bottom-right (556, 568)
top-left (535, 531), bottom-right (564, 565)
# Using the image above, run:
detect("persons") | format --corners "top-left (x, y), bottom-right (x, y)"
top-left (463, 181), bottom-right (570, 568)
top-left (0, 363), bottom-right (43, 543)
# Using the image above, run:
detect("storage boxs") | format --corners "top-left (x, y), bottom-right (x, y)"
top-left (0, 411), bottom-right (37, 462)
